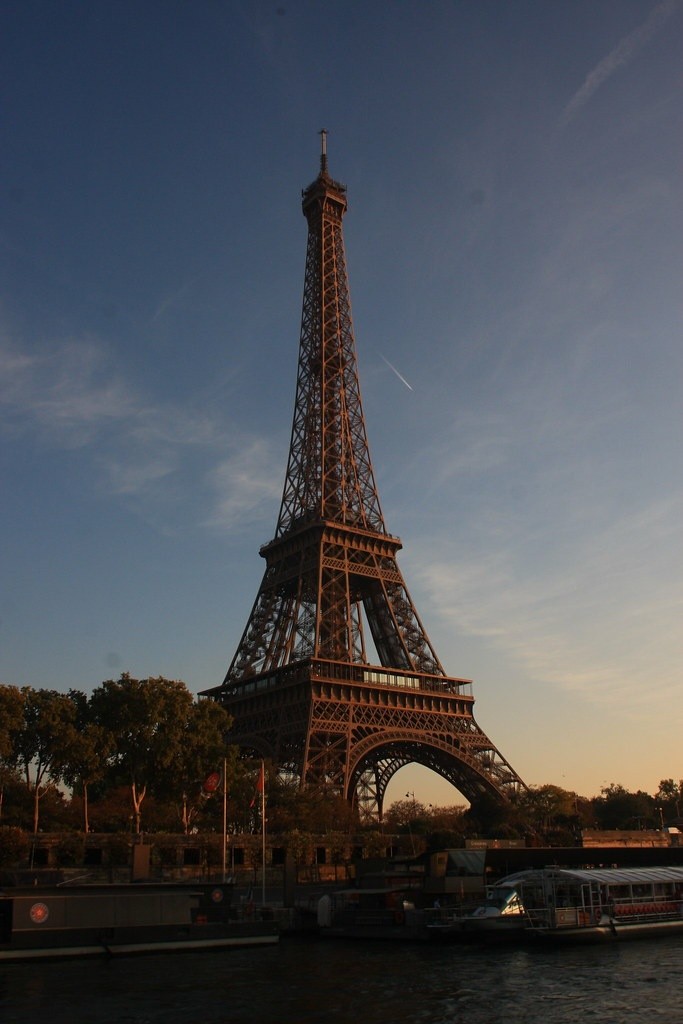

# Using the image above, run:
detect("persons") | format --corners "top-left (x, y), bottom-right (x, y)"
top-left (607, 896), bottom-right (616, 918)
top-left (433, 897), bottom-right (441, 920)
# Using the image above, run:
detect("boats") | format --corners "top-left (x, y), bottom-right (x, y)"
top-left (289, 884), bottom-right (490, 939)
top-left (460, 864), bottom-right (683, 944)
top-left (0, 881), bottom-right (298, 960)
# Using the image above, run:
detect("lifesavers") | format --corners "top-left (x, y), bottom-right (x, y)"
top-left (595, 907), bottom-right (604, 921)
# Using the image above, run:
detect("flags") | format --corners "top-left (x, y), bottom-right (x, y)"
top-left (255, 766), bottom-right (263, 793)
